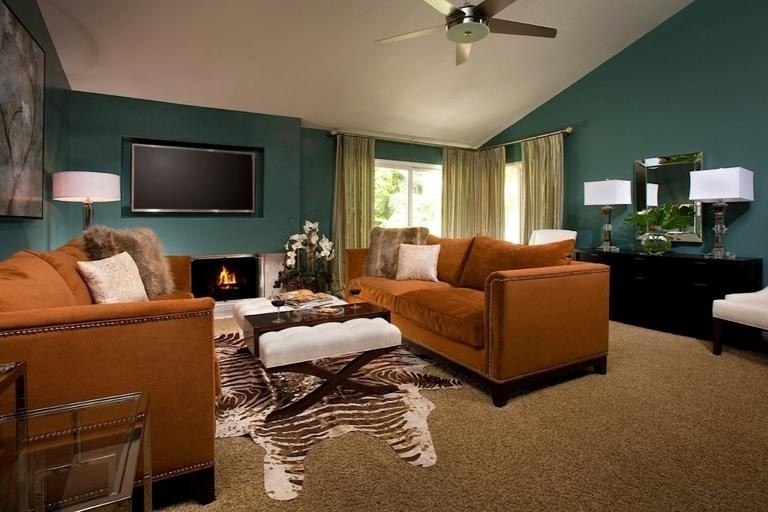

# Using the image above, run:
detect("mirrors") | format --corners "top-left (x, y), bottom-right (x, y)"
top-left (635, 154), bottom-right (702, 245)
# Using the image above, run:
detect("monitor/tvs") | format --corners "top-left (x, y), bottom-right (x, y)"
top-left (130, 141), bottom-right (256, 216)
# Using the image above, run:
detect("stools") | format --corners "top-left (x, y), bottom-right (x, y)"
top-left (711, 288), bottom-right (768, 355)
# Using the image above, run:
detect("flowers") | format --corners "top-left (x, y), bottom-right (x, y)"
top-left (284, 218), bottom-right (335, 268)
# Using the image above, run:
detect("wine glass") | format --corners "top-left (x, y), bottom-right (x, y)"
top-left (348, 279), bottom-right (363, 310)
top-left (271, 288), bottom-right (287, 324)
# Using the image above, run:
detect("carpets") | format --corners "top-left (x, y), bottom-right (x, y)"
top-left (216, 333), bottom-right (463, 501)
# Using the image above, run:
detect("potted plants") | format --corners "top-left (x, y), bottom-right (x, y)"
top-left (625, 201), bottom-right (693, 255)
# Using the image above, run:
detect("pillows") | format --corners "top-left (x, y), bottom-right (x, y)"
top-left (461, 233), bottom-right (574, 289)
top-left (85, 226), bottom-right (176, 299)
top-left (77, 250), bottom-right (148, 305)
top-left (395, 244), bottom-right (439, 284)
top-left (423, 235), bottom-right (470, 285)
top-left (113, 302), bottom-right (114, 304)
top-left (360, 226), bottom-right (430, 277)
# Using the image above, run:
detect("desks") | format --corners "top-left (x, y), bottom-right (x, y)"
top-left (2, 358), bottom-right (154, 512)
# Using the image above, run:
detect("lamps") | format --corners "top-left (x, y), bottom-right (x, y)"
top-left (52, 170), bottom-right (122, 231)
top-left (646, 182), bottom-right (659, 209)
top-left (690, 167), bottom-right (755, 259)
top-left (583, 179), bottom-right (631, 252)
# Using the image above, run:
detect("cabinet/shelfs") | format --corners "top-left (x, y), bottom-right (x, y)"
top-left (575, 249), bottom-right (764, 355)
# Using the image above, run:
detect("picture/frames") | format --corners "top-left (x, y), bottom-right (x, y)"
top-left (1, 25), bottom-right (46, 220)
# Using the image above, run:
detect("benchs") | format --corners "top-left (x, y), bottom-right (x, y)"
top-left (234, 294), bottom-right (349, 355)
top-left (258, 316), bottom-right (404, 423)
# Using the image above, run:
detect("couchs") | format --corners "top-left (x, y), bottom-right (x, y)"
top-left (0, 237), bottom-right (217, 506)
top-left (343, 247), bottom-right (611, 409)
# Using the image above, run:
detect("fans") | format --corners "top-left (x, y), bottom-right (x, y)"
top-left (371, 1), bottom-right (555, 66)
